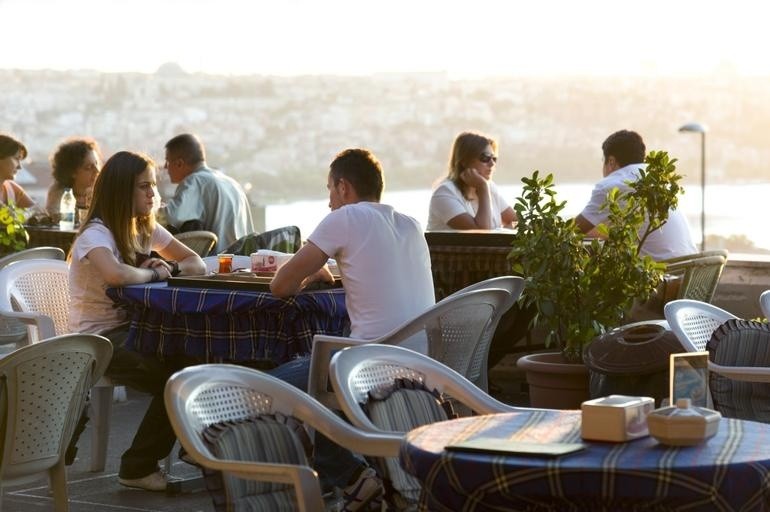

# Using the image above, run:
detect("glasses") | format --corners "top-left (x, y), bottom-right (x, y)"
top-left (479, 152), bottom-right (497, 164)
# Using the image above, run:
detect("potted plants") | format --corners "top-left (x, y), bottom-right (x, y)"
top-left (501, 146), bottom-right (690, 411)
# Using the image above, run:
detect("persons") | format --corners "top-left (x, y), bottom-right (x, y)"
top-left (573, 129), bottom-right (699, 322)
top-left (64, 152), bottom-right (208, 493)
top-left (424, 131), bottom-right (519, 232)
top-left (151, 133), bottom-right (255, 256)
top-left (0, 134), bottom-right (57, 227)
top-left (265, 147), bottom-right (437, 512)
top-left (44, 139), bottom-right (104, 223)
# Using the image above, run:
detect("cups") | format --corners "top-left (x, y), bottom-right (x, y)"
top-left (512, 220), bottom-right (529, 229)
top-left (78, 208), bottom-right (88, 228)
top-left (217, 253), bottom-right (234, 274)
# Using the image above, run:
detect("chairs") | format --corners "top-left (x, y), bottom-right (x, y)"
top-left (437, 272), bottom-right (527, 396)
top-left (666, 297), bottom-right (769, 423)
top-left (655, 247), bottom-right (731, 305)
top-left (759, 287), bottom-right (769, 322)
top-left (326, 342), bottom-right (532, 511)
top-left (305, 286), bottom-right (513, 502)
top-left (161, 361), bottom-right (410, 511)
top-left (1, 208), bottom-right (219, 509)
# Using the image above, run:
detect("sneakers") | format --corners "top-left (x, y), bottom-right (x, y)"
top-left (119, 467), bottom-right (183, 492)
top-left (339, 469), bottom-right (382, 512)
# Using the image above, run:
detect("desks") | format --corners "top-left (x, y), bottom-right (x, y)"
top-left (99, 279), bottom-right (352, 390)
top-left (397, 408), bottom-right (768, 510)
top-left (424, 226), bottom-right (604, 373)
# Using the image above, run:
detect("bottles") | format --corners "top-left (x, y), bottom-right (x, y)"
top-left (647, 397), bottom-right (722, 447)
top-left (59, 187), bottom-right (76, 231)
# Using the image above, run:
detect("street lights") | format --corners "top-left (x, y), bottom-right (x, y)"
top-left (677, 123), bottom-right (706, 252)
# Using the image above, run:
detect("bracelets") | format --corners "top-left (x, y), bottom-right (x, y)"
top-left (165, 260), bottom-right (179, 277)
top-left (149, 267), bottom-right (159, 282)
